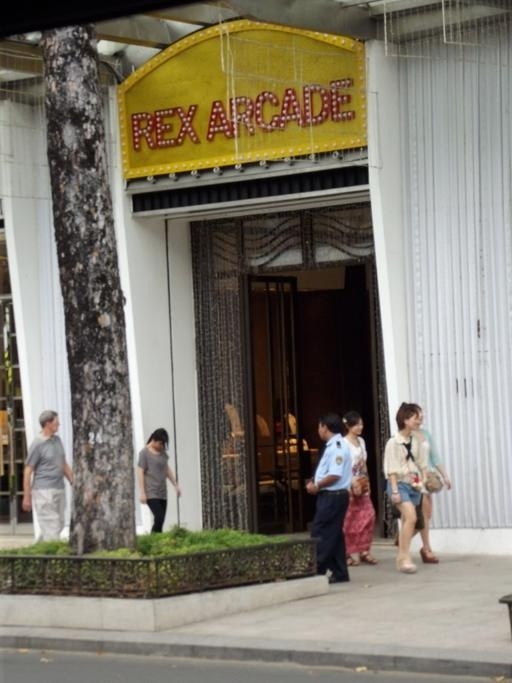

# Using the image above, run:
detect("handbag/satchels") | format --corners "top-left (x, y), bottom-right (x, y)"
top-left (425, 472), bottom-right (443, 493)
top-left (394, 493), bottom-right (425, 530)
top-left (351, 475), bottom-right (371, 498)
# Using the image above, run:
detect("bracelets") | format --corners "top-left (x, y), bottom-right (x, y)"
top-left (392, 490), bottom-right (400, 494)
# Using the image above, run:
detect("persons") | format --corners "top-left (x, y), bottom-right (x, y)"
top-left (305, 413), bottom-right (350, 584)
top-left (136, 427), bottom-right (181, 532)
top-left (394, 401), bottom-right (455, 563)
top-left (382, 404), bottom-right (427, 574)
top-left (342, 409), bottom-right (378, 566)
top-left (20, 408), bottom-right (75, 544)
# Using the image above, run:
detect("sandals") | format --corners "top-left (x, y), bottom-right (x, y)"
top-left (420, 548), bottom-right (439, 564)
top-left (396, 555), bottom-right (418, 574)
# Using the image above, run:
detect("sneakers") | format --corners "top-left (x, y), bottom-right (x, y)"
top-left (315, 550), bottom-right (377, 584)
